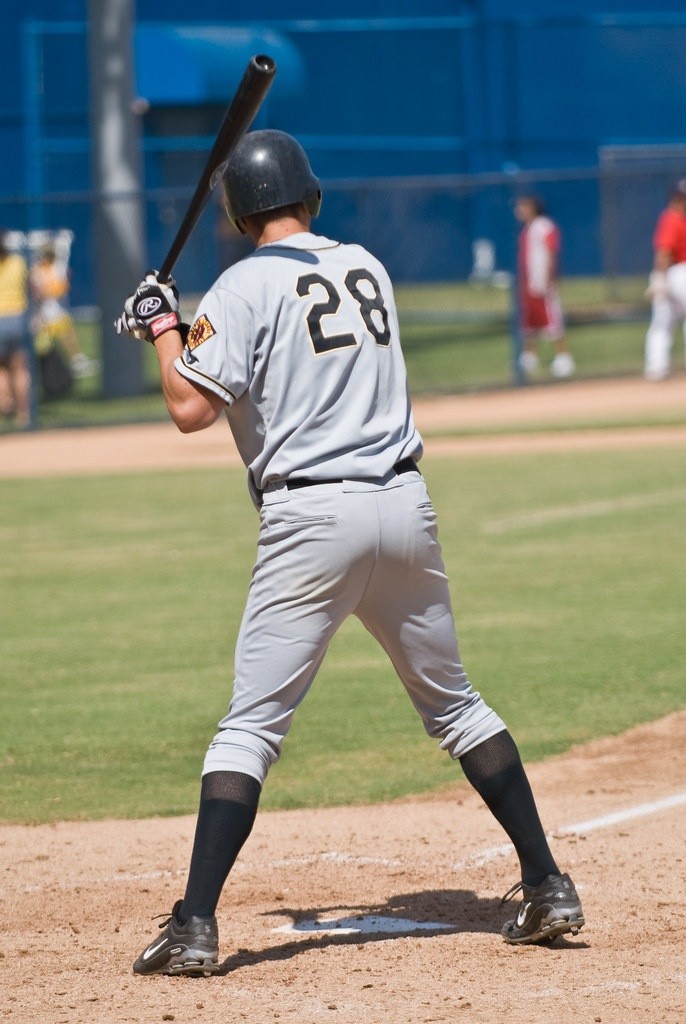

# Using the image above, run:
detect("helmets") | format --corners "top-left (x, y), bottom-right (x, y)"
top-left (221, 129), bottom-right (322, 236)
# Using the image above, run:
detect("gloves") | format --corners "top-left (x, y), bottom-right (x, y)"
top-left (124, 270), bottom-right (180, 345)
top-left (113, 311), bottom-right (191, 348)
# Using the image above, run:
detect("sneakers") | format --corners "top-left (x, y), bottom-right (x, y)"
top-left (499, 873), bottom-right (584, 944)
top-left (133, 900), bottom-right (220, 975)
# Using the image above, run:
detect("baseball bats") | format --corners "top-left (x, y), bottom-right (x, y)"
top-left (155, 52), bottom-right (280, 284)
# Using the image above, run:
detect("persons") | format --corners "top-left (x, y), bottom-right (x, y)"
top-left (0, 228), bottom-right (97, 432)
top-left (113, 130), bottom-right (587, 975)
top-left (643, 181), bottom-right (686, 384)
top-left (510, 194), bottom-right (578, 381)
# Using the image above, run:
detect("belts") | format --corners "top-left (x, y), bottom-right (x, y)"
top-left (285, 457), bottom-right (421, 490)
